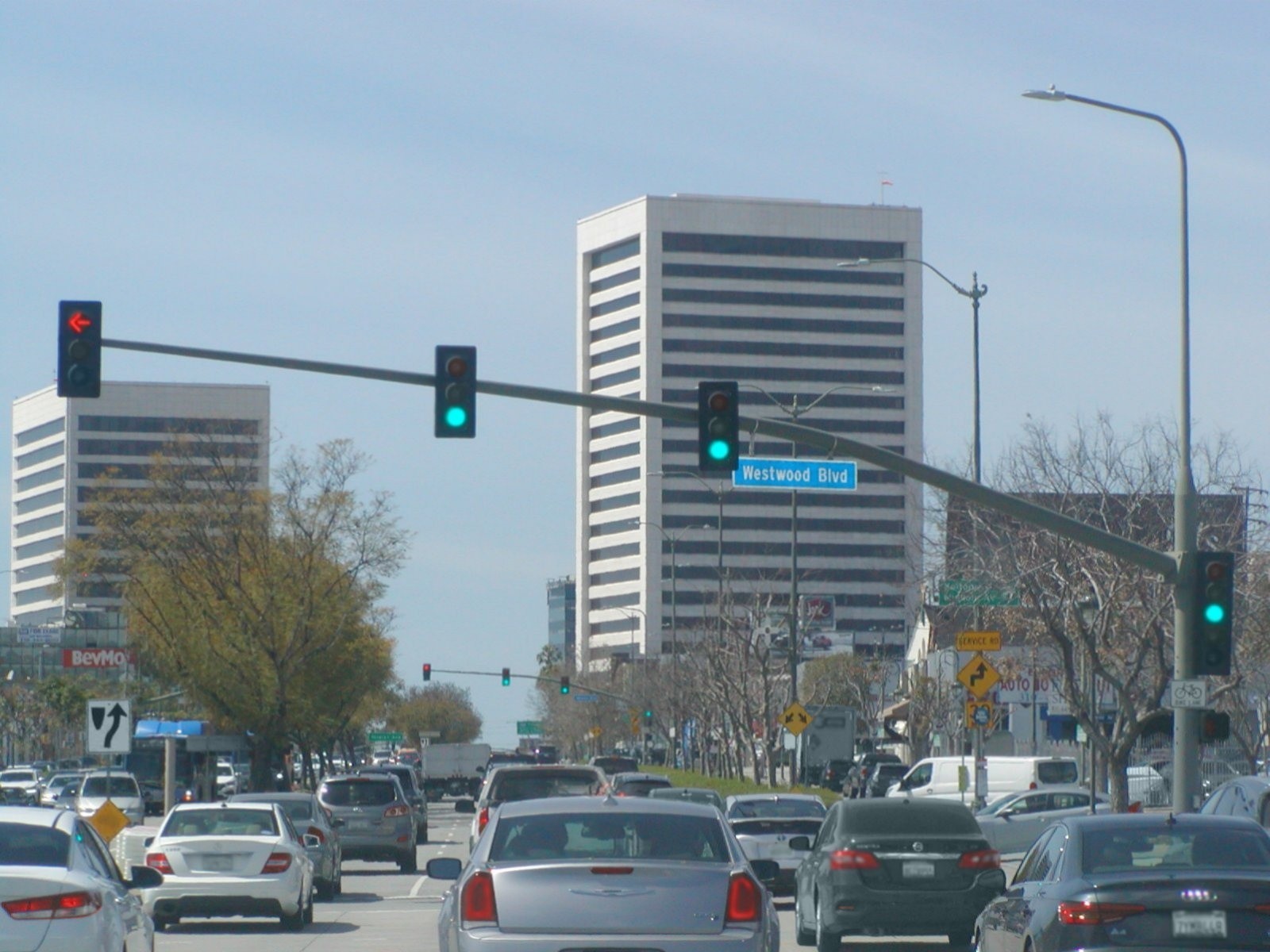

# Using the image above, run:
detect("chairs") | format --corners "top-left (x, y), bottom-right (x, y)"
top-left (1188, 833), bottom-right (1229, 866)
top-left (626, 813), bottom-right (681, 858)
top-left (219, 818), bottom-right (264, 834)
top-left (179, 818), bottom-right (203, 837)
top-left (1097, 841), bottom-right (1134, 869)
top-left (513, 821), bottom-right (572, 857)
top-left (791, 803), bottom-right (817, 817)
top-left (757, 804), bottom-right (777, 819)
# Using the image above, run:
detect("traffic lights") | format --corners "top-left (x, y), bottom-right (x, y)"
top-left (1190, 550), bottom-right (1239, 683)
top-left (423, 663), bottom-right (431, 681)
top-left (502, 668), bottom-right (511, 686)
top-left (694, 379), bottom-right (742, 479)
top-left (643, 703), bottom-right (654, 727)
top-left (432, 344), bottom-right (479, 439)
top-left (56, 299), bottom-right (104, 401)
top-left (560, 676), bottom-right (571, 695)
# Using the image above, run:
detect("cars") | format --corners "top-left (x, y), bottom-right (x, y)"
top-left (455, 762), bottom-right (621, 856)
top-left (0, 746), bottom-right (565, 830)
top-left (355, 763), bottom-right (433, 844)
top-left (424, 808), bottom-right (785, 952)
top-left (969, 811), bottom-right (1269, 952)
top-left (214, 792), bottom-right (347, 902)
top-left (312, 772), bottom-right (424, 876)
top-left (586, 753), bottom-right (1269, 952)
top-left (426, 794), bottom-right (781, 950)
top-left (142, 802), bottom-right (321, 936)
top-left (0, 805), bottom-right (167, 952)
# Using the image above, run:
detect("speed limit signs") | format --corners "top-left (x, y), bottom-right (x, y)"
top-left (965, 701), bottom-right (997, 735)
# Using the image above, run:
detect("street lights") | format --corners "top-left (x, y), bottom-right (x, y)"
top-left (736, 383), bottom-right (899, 790)
top-left (838, 258), bottom-right (985, 810)
top-left (635, 520), bottom-right (710, 771)
top-left (646, 468), bottom-right (735, 675)
top-left (1021, 87), bottom-right (1199, 824)
